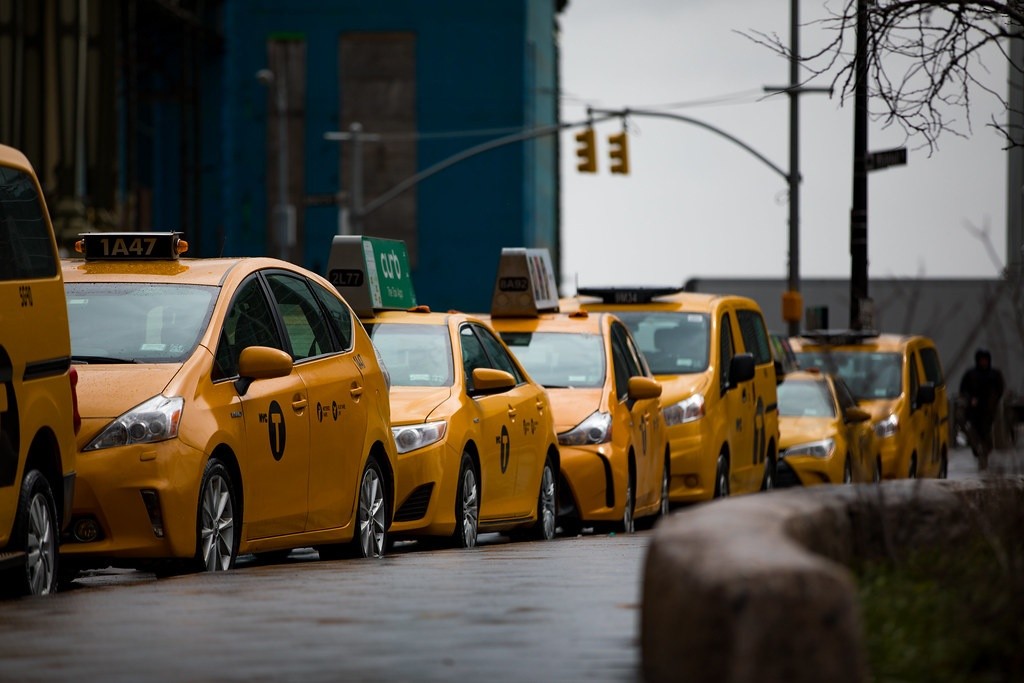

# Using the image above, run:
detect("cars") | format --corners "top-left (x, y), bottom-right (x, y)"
top-left (313, 233), bottom-right (559, 551)
top-left (51, 230), bottom-right (400, 579)
top-left (759, 333), bottom-right (882, 499)
top-left (467, 246), bottom-right (674, 532)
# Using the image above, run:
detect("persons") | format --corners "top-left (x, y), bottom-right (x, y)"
top-left (958, 349), bottom-right (1004, 469)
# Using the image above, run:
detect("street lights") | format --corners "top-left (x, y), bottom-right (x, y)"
top-left (257, 67), bottom-right (294, 262)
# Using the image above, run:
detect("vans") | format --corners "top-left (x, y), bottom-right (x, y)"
top-left (0, 145), bottom-right (80, 604)
top-left (811, 324), bottom-right (950, 484)
top-left (567, 241), bottom-right (781, 514)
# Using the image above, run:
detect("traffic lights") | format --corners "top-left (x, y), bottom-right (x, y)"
top-left (609, 131), bottom-right (629, 174)
top-left (576, 127), bottom-right (596, 172)
